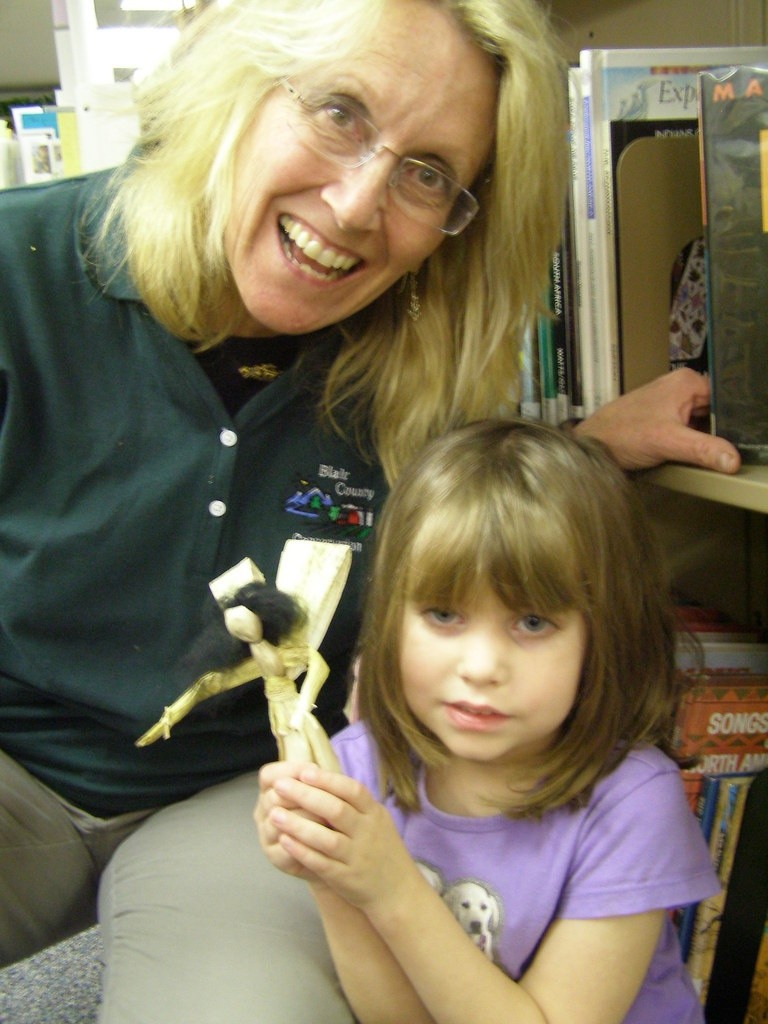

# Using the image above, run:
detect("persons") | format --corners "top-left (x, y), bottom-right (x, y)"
top-left (135, 536), bottom-right (342, 770)
top-left (0, 0), bottom-right (739, 1024)
top-left (255, 416), bottom-right (724, 1024)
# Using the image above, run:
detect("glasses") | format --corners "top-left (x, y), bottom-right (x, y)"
top-left (276, 76), bottom-right (481, 236)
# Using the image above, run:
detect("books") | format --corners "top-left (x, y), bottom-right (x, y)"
top-left (516, 45), bottom-right (767, 466)
top-left (0, 105), bottom-right (129, 191)
top-left (652, 601), bottom-right (767, 1024)
